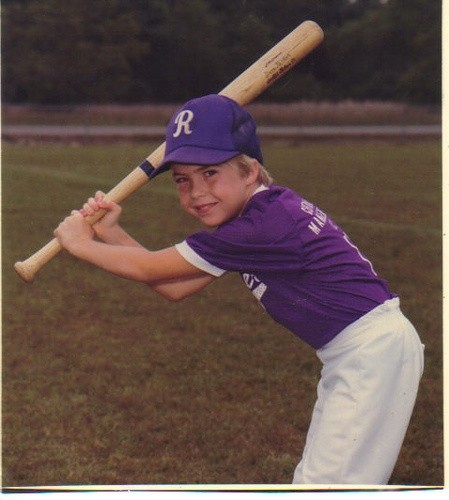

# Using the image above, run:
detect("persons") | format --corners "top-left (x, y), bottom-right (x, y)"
top-left (52, 94), bottom-right (425, 484)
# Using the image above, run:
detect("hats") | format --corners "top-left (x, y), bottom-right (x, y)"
top-left (149, 94), bottom-right (264, 176)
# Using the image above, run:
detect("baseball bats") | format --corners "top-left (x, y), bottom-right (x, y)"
top-left (14, 20), bottom-right (325, 282)
